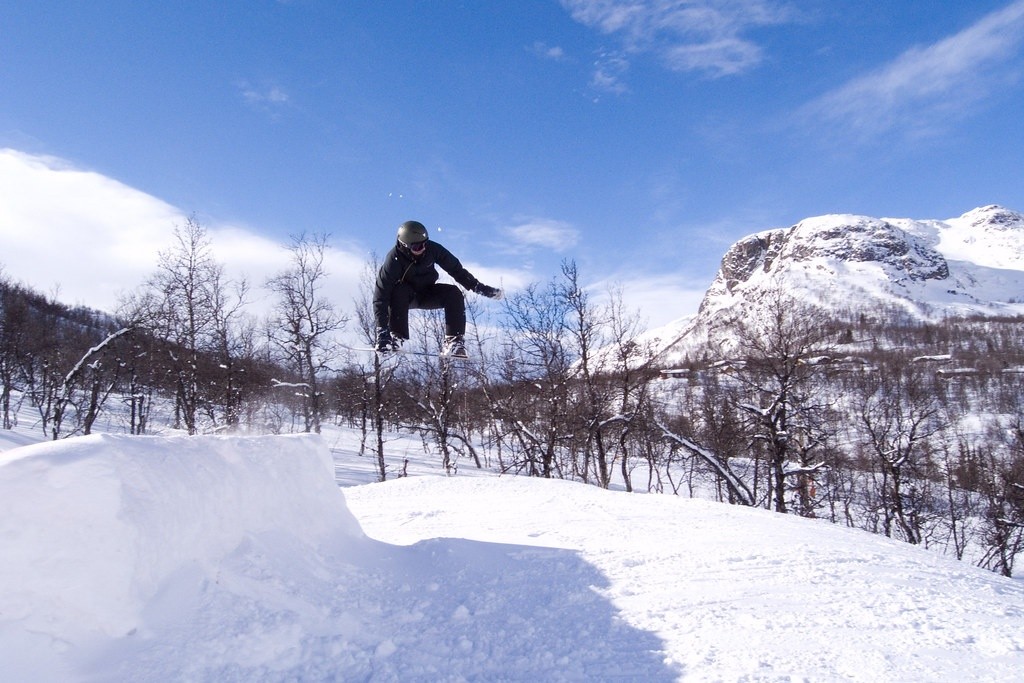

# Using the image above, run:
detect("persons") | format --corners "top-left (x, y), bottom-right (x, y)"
top-left (372, 220), bottom-right (502, 359)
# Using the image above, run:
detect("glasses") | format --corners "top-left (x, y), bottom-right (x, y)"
top-left (411, 238), bottom-right (428, 251)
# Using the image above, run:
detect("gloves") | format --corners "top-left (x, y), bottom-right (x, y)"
top-left (474, 282), bottom-right (501, 300)
top-left (376, 327), bottom-right (392, 352)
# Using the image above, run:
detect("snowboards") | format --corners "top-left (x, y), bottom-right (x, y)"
top-left (329, 338), bottom-right (471, 364)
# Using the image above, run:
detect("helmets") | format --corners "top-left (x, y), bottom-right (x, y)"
top-left (397, 221), bottom-right (429, 247)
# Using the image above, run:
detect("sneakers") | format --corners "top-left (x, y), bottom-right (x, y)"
top-left (389, 332), bottom-right (404, 351)
top-left (444, 337), bottom-right (467, 357)
